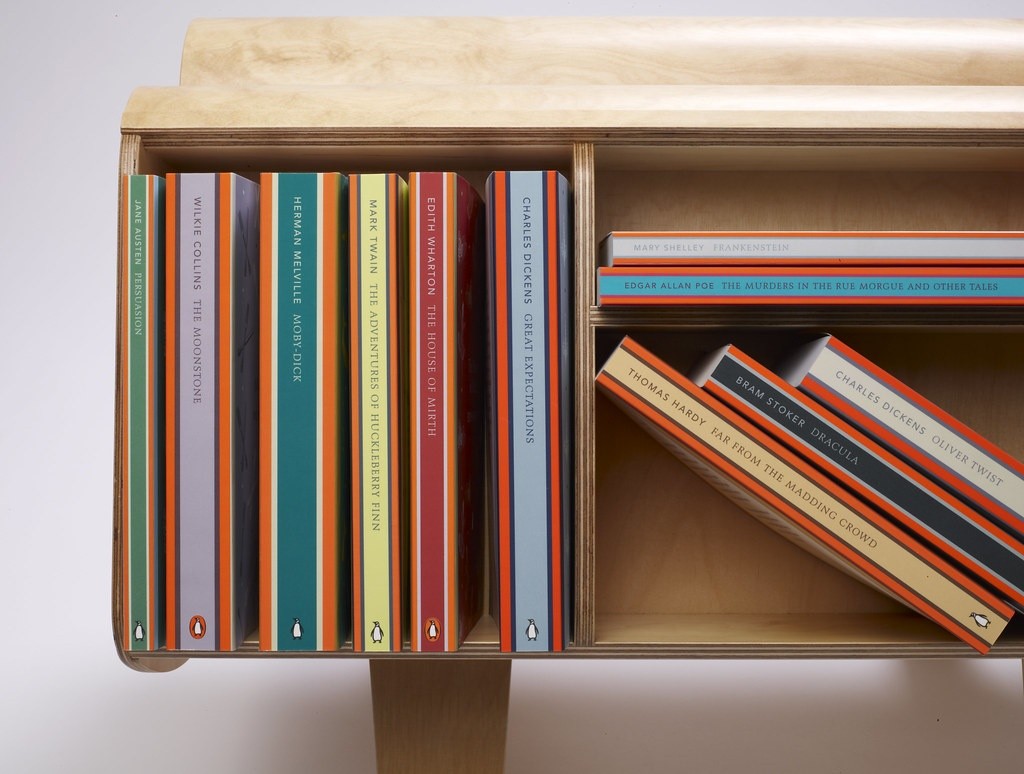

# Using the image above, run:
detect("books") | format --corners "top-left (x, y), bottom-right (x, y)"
top-left (595, 331), bottom-right (1024, 656)
top-left (597, 232), bottom-right (1024, 309)
top-left (117, 170), bottom-right (570, 651)
